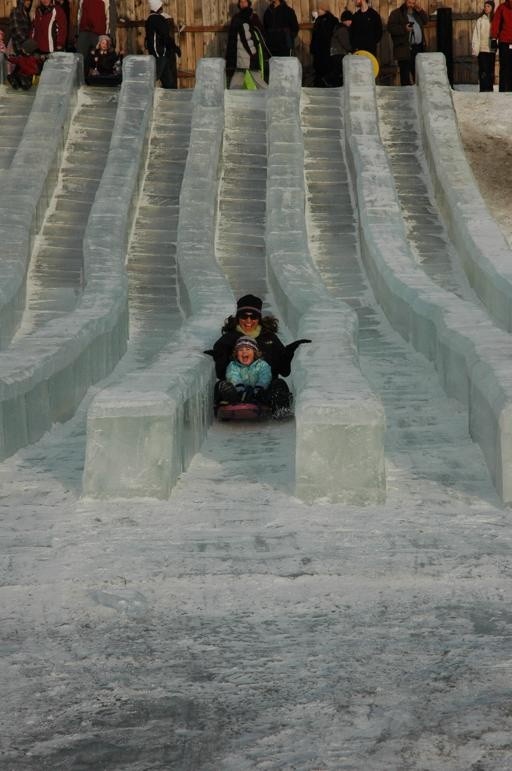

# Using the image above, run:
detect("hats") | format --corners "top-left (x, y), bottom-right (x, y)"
top-left (316, 1), bottom-right (329, 11)
top-left (237, 295), bottom-right (262, 316)
top-left (340, 10), bottom-right (354, 21)
top-left (149, 0), bottom-right (164, 12)
top-left (23, 40), bottom-right (38, 54)
top-left (235, 335), bottom-right (259, 352)
top-left (96, 35), bottom-right (111, 51)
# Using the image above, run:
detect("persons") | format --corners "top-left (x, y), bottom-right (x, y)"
top-left (0, 0), bottom-right (181, 90)
top-left (203, 294), bottom-right (312, 420)
top-left (472, 0), bottom-right (497, 92)
top-left (225, 0), bottom-right (383, 90)
top-left (490, 0), bottom-right (512, 92)
top-left (386, 0), bottom-right (428, 86)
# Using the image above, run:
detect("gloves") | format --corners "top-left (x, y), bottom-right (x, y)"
top-left (204, 350), bottom-right (214, 356)
top-left (235, 383), bottom-right (245, 397)
top-left (287, 339), bottom-right (311, 350)
top-left (491, 39), bottom-right (499, 49)
top-left (253, 386), bottom-right (265, 398)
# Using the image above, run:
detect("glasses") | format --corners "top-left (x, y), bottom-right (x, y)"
top-left (238, 313), bottom-right (259, 321)
